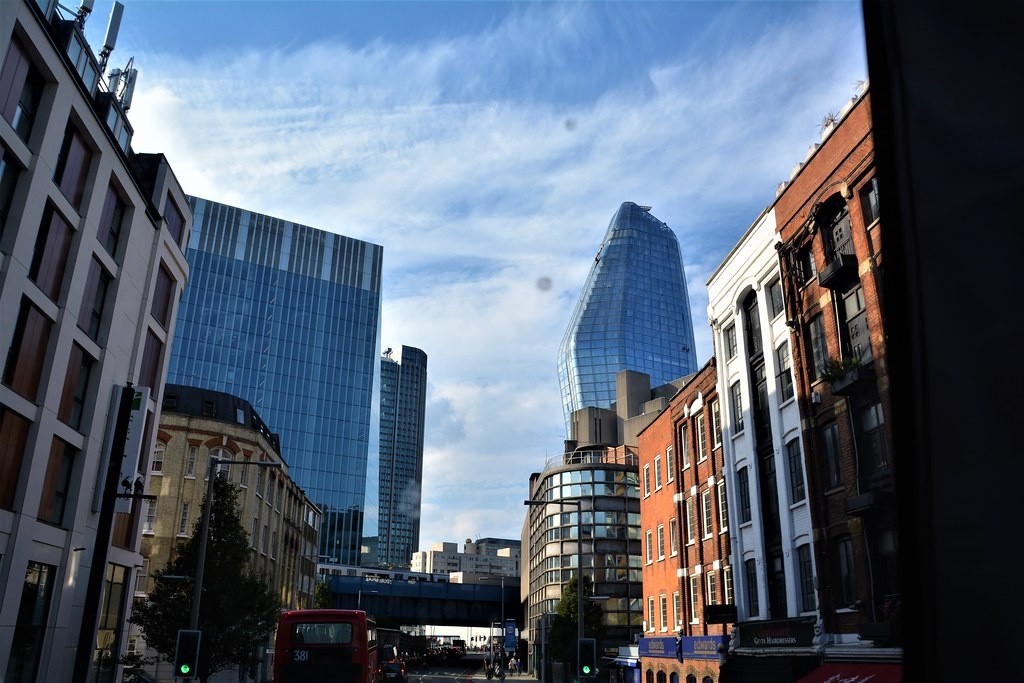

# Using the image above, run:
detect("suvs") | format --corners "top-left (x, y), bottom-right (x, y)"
top-left (379, 660), bottom-right (408, 683)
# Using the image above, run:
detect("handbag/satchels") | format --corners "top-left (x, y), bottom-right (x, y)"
top-left (508, 665), bottom-right (512, 669)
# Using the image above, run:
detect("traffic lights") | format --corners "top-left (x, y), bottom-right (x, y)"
top-left (173, 629), bottom-right (201, 678)
top-left (578, 638), bottom-right (596, 677)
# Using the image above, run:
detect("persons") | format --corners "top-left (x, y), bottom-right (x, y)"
top-left (511, 657), bottom-right (516, 669)
top-left (486, 663), bottom-right (500, 679)
top-left (516, 658), bottom-right (523, 676)
top-left (508, 660), bottom-right (515, 676)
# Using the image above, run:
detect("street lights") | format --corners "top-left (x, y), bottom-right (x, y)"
top-left (189, 457), bottom-right (283, 627)
top-left (356, 590), bottom-right (378, 609)
top-left (524, 500), bottom-right (584, 642)
top-left (479, 576), bottom-right (506, 681)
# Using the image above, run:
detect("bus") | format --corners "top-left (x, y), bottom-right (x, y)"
top-left (273, 610), bottom-right (380, 683)
